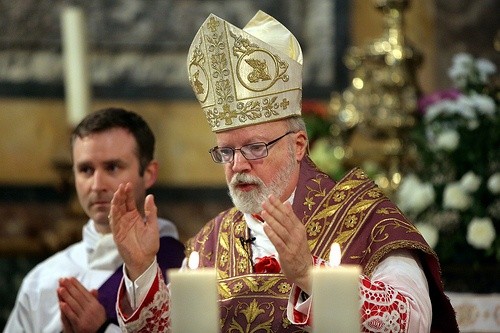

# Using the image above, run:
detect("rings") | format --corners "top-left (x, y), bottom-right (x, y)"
top-left (107, 214), bottom-right (111, 219)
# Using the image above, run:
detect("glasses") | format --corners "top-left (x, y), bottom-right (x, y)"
top-left (209, 130), bottom-right (294, 164)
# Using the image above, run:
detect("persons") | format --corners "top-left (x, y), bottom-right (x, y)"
top-left (2, 107), bottom-right (183, 333)
top-left (109, 52), bottom-right (432, 333)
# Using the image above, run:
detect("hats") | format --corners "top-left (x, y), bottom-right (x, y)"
top-left (186, 9), bottom-right (303, 132)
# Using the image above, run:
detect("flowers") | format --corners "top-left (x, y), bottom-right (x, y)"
top-left (393, 52), bottom-right (499, 294)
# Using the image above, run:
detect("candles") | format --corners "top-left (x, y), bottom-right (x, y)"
top-left (166, 251), bottom-right (220, 333)
top-left (309, 243), bottom-right (362, 333)
